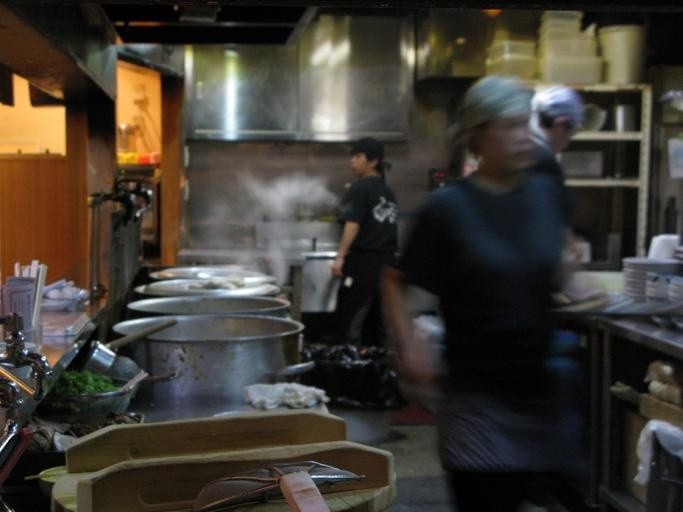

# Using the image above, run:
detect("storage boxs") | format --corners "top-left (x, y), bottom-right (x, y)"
top-left (485, 10), bottom-right (603, 83)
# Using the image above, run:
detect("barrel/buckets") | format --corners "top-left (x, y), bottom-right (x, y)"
top-left (598, 23), bottom-right (646, 85)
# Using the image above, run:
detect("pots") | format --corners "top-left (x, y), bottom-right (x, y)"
top-left (78, 265), bottom-right (315, 422)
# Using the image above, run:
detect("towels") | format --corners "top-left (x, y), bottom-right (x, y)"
top-left (242, 383), bottom-right (330, 409)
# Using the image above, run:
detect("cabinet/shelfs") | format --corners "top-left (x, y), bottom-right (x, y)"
top-left (527, 83), bottom-right (651, 256)
top-left (568, 312), bottom-right (683, 512)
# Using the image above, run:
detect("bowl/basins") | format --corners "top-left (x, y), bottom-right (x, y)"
top-left (600, 24), bottom-right (645, 85)
top-left (83, 341), bottom-right (117, 372)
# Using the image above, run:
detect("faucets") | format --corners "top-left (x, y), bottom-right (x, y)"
top-left (86, 174), bottom-right (153, 298)
top-left (0, 311), bottom-right (53, 380)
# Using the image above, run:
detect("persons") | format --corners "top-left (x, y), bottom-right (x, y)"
top-left (329, 137), bottom-right (399, 347)
top-left (394, 72), bottom-right (610, 512)
top-left (527, 81), bottom-right (587, 224)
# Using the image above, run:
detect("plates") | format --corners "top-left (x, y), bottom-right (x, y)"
top-left (621, 257), bottom-right (682, 333)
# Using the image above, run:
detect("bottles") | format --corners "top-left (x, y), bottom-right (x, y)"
top-left (616, 103), bottom-right (634, 135)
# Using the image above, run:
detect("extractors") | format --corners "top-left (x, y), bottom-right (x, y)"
top-left (188, 10), bottom-right (410, 153)
